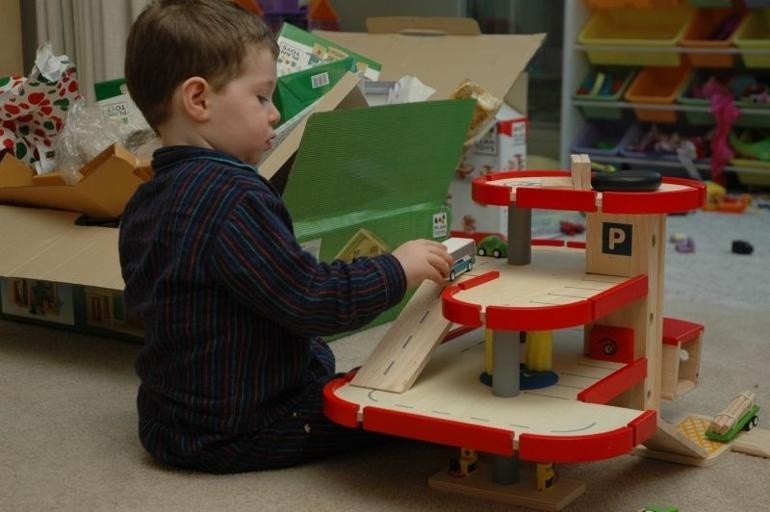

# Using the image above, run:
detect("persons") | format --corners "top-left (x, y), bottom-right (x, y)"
top-left (119, 0), bottom-right (452, 474)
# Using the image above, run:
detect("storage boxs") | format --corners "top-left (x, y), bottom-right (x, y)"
top-left (736, 7), bottom-right (770, 72)
top-left (733, 74), bottom-right (768, 126)
top-left (679, 3), bottom-right (749, 68)
top-left (725, 152), bottom-right (770, 189)
top-left (623, 67), bottom-right (681, 125)
top-left (570, 119), bottom-right (634, 159)
top-left (574, 9), bottom-right (684, 68)
top-left (574, 66), bottom-right (637, 121)
top-left (446, 73), bottom-right (527, 244)
top-left (0, 26), bottom-right (552, 344)
top-left (676, 71), bottom-right (732, 124)
top-left (622, 122), bottom-right (718, 178)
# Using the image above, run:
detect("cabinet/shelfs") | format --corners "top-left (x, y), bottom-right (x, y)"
top-left (560, 0), bottom-right (770, 193)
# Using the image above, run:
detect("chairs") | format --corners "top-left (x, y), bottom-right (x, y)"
top-left (366, 17), bottom-right (481, 35)
top-left (257, 1), bottom-right (307, 29)
